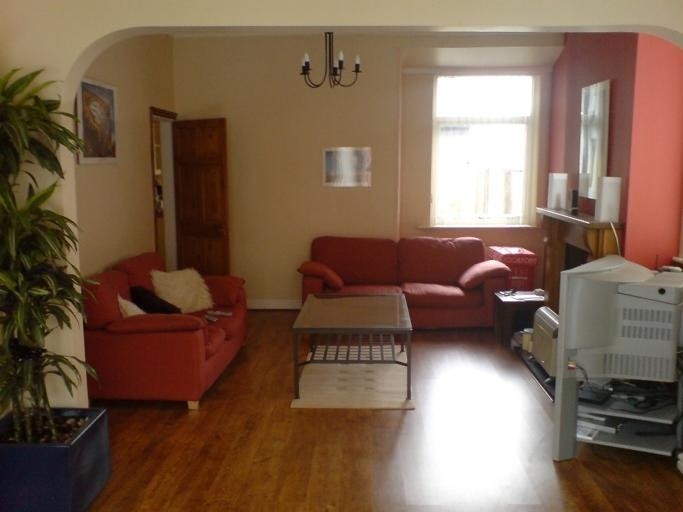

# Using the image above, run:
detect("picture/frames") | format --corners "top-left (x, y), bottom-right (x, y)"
top-left (73, 73), bottom-right (121, 166)
top-left (320, 146), bottom-right (373, 190)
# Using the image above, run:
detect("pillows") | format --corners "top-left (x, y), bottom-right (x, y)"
top-left (150, 267), bottom-right (214, 314)
top-left (128, 283), bottom-right (180, 314)
top-left (112, 292), bottom-right (146, 318)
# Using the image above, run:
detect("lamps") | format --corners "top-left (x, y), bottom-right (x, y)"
top-left (544, 169), bottom-right (575, 213)
top-left (296, 29), bottom-right (364, 92)
top-left (594, 174), bottom-right (623, 224)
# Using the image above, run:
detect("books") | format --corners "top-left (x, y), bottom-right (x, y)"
top-left (578, 410), bottom-right (607, 422)
top-left (576, 426), bottom-right (600, 441)
top-left (577, 419), bottom-right (626, 436)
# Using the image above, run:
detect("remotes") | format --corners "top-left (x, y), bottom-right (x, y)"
top-left (207, 311), bottom-right (232, 316)
top-left (205, 315), bottom-right (219, 322)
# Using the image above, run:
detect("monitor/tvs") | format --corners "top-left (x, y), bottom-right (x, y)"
top-left (556, 255), bottom-right (683, 383)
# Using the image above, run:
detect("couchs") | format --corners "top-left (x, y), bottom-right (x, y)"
top-left (78, 246), bottom-right (254, 419)
top-left (295, 232), bottom-right (512, 334)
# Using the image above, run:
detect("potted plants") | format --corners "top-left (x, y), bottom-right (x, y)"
top-left (0, 62), bottom-right (117, 512)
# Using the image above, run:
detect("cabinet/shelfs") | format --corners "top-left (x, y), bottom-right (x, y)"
top-left (545, 370), bottom-right (680, 479)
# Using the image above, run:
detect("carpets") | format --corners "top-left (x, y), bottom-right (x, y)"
top-left (290, 340), bottom-right (416, 413)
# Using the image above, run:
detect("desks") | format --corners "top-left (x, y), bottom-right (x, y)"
top-left (287, 290), bottom-right (415, 405)
top-left (491, 286), bottom-right (547, 350)
